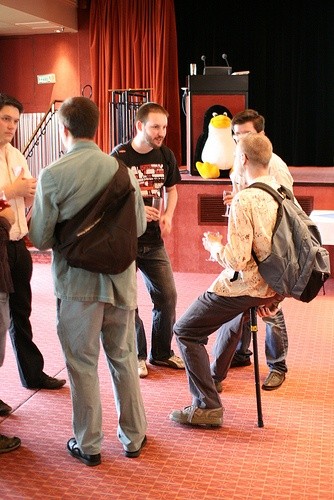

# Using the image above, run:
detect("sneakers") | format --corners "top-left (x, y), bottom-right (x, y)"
top-left (0, 434), bottom-right (22, 453)
top-left (124, 435), bottom-right (148, 458)
top-left (148, 350), bottom-right (187, 370)
top-left (135, 356), bottom-right (149, 379)
top-left (67, 436), bottom-right (102, 467)
top-left (169, 400), bottom-right (223, 427)
top-left (23, 373), bottom-right (66, 390)
top-left (229, 350), bottom-right (251, 367)
top-left (262, 368), bottom-right (286, 390)
top-left (0, 401), bottom-right (12, 415)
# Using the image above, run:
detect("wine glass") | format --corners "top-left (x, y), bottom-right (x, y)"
top-left (205, 231), bottom-right (221, 262)
top-left (221, 191), bottom-right (233, 217)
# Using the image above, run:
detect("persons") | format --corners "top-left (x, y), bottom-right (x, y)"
top-left (25, 97), bottom-right (148, 467)
top-left (167, 131), bottom-right (301, 425)
top-left (1, 93), bottom-right (67, 413)
top-left (0, 193), bottom-right (22, 453)
top-left (105, 101), bottom-right (187, 378)
top-left (221, 110), bottom-right (296, 390)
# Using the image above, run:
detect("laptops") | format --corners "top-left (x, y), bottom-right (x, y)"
top-left (203, 66), bottom-right (232, 76)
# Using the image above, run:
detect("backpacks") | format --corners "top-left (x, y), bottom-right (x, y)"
top-left (231, 181), bottom-right (330, 303)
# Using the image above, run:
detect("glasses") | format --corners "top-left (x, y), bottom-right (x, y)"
top-left (231, 132), bottom-right (249, 141)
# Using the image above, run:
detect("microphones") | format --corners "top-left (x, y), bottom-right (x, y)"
top-left (201, 55), bottom-right (206, 66)
top-left (222, 54), bottom-right (229, 67)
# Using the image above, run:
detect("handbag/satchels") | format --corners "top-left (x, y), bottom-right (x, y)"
top-left (51, 158), bottom-right (141, 276)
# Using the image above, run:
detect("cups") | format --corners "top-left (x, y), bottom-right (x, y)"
top-left (152, 197), bottom-right (163, 221)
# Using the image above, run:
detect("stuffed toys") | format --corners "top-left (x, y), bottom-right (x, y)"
top-left (194, 105), bottom-right (236, 180)
